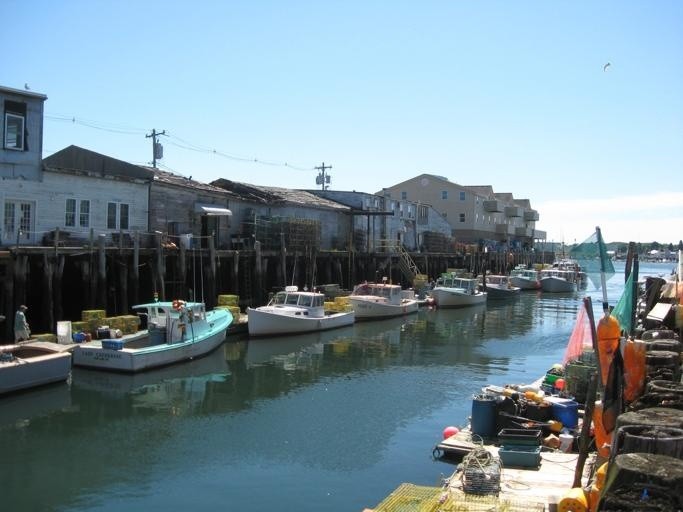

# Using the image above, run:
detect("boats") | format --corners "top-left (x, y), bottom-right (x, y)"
top-left (72, 299), bottom-right (234, 372)
top-left (0, 339), bottom-right (73, 393)
top-left (340, 256), bottom-right (591, 315)
top-left (246, 289), bottom-right (356, 334)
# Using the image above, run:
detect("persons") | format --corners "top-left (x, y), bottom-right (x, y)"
top-left (12, 304), bottom-right (30, 343)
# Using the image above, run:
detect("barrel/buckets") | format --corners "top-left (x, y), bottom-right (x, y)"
top-left (472, 396), bottom-right (496, 436)
top-left (57, 321), bottom-right (72, 344)
top-left (553, 401), bottom-right (578, 427)
